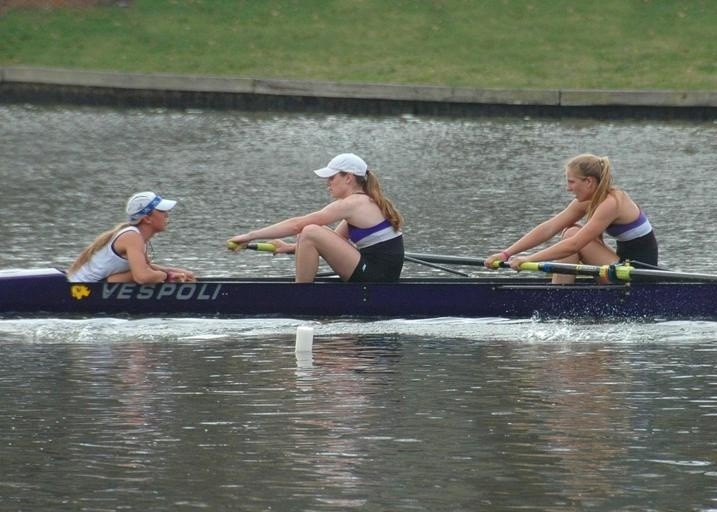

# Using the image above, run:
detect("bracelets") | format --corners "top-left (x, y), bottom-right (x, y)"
top-left (164, 271), bottom-right (173, 282)
top-left (501, 251), bottom-right (509, 261)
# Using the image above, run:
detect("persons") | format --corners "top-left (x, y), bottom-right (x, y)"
top-left (230, 151), bottom-right (405, 284)
top-left (483, 154), bottom-right (658, 284)
top-left (68, 191), bottom-right (196, 285)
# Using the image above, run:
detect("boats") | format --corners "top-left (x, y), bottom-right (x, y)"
top-left (1, 267), bottom-right (715, 323)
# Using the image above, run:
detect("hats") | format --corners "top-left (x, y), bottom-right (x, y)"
top-left (313, 152), bottom-right (368, 178)
top-left (125, 191), bottom-right (178, 225)
